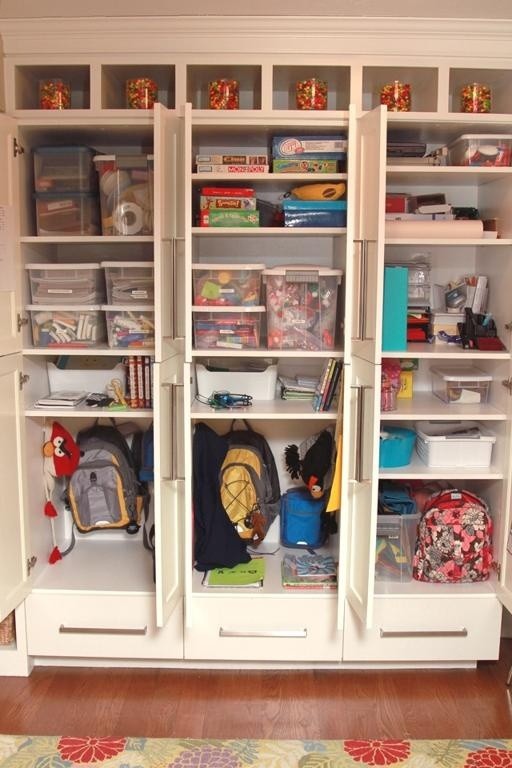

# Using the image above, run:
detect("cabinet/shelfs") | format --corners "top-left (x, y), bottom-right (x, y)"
top-left (0, 16), bottom-right (512, 677)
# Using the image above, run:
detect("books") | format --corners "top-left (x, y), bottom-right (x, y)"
top-left (278, 358), bottom-right (343, 413)
top-left (280, 554), bottom-right (338, 591)
top-left (128, 355), bottom-right (155, 409)
top-left (33, 390), bottom-right (92, 409)
top-left (200, 555), bottom-right (265, 589)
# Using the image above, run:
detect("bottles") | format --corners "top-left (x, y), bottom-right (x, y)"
top-left (296, 77), bottom-right (328, 110)
top-left (207, 78), bottom-right (241, 110)
top-left (381, 78), bottom-right (414, 111)
top-left (41, 77), bottom-right (72, 109)
top-left (459, 77), bottom-right (494, 110)
top-left (127, 78), bottom-right (159, 109)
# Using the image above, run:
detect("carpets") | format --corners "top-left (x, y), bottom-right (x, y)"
top-left (0, 735), bottom-right (512, 768)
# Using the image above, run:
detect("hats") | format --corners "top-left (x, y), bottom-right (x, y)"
top-left (44, 420), bottom-right (80, 564)
top-left (286, 427), bottom-right (336, 497)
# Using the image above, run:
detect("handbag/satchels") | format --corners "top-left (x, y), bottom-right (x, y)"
top-left (280, 489), bottom-right (331, 549)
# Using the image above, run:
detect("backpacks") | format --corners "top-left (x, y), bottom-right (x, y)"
top-left (414, 488), bottom-right (494, 583)
top-left (217, 420), bottom-right (280, 546)
top-left (68, 418), bottom-right (143, 534)
